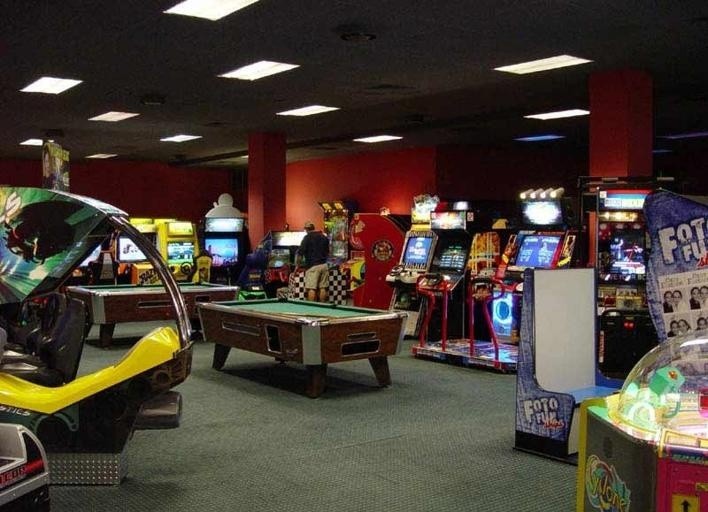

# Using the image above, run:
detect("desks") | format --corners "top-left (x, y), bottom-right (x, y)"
top-left (196, 294), bottom-right (408, 397)
top-left (62, 280), bottom-right (240, 349)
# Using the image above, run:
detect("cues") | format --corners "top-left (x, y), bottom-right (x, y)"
top-left (239, 308), bottom-right (337, 319)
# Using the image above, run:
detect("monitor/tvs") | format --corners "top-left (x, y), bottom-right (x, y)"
top-left (167, 241), bottom-right (194, 261)
top-left (515, 235), bottom-right (561, 267)
top-left (402, 235), bottom-right (433, 266)
top-left (609, 228), bottom-right (646, 275)
top-left (118, 235), bottom-right (147, 261)
top-left (438, 241), bottom-right (468, 269)
top-left (267, 248), bottom-right (291, 269)
top-left (205, 238), bottom-right (239, 267)
top-left (76, 242), bottom-right (101, 267)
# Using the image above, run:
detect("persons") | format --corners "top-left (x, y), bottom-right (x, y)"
top-left (662, 284), bottom-right (708, 337)
top-left (293, 220), bottom-right (329, 302)
